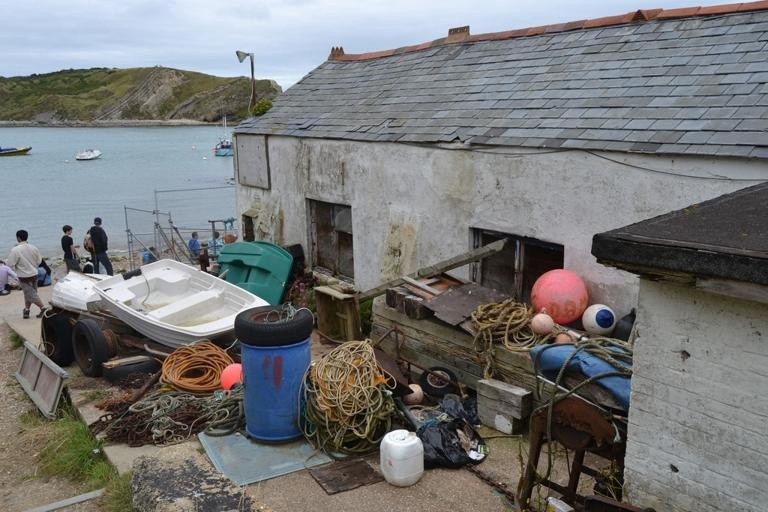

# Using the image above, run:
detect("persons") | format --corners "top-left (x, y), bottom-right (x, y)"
top-left (189, 231), bottom-right (201, 257)
top-left (142, 248), bottom-right (149, 265)
top-left (149, 247), bottom-right (159, 263)
top-left (61, 225), bottom-right (82, 274)
top-left (0, 261), bottom-right (17, 295)
top-left (207, 232), bottom-right (225, 267)
top-left (87, 217), bottom-right (113, 276)
top-left (37, 257), bottom-right (52, 286)
top-left (7, 230), bottom-right (48, 319)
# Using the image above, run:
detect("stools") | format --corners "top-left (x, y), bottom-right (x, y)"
top-left (514, 395), bottom-right (628, 512)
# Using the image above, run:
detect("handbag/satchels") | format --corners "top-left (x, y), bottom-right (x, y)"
top-left (83, 234), bottom-right (96, 254)
top-left (37, 280), bottom-right (44, 287)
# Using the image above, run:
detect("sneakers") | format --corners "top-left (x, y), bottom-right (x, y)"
top-left (22, 307), bottom-right (48, 319)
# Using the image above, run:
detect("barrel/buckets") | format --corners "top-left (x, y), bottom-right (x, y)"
top-left (239, 339), bottom-right (312, 442)
top-left (381, 427), bottom-right (425, 486)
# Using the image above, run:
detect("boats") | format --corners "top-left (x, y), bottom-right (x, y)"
top-left (214, 116), bottom-right (233, 156)
top-left (0, 145), bottom-right (32, 156)
top-left (76, 149), bottom-right (102, 159)
top-left (48, 241), bottom-right (294, 349)
